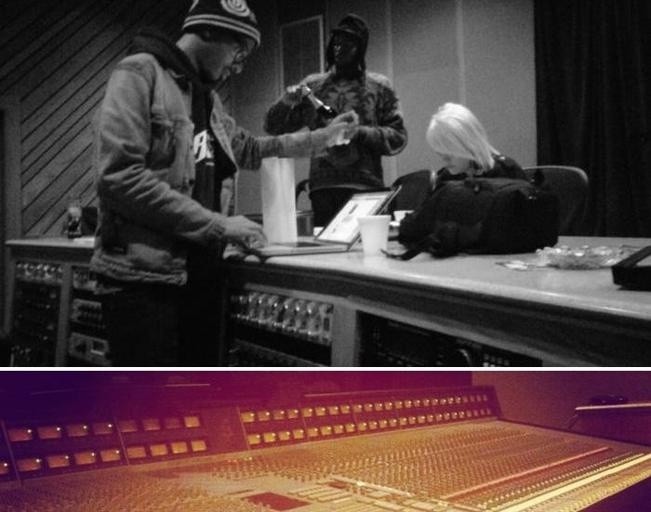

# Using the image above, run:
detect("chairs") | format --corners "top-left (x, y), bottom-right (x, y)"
top-left (380, 166), bottom-right (590, 237)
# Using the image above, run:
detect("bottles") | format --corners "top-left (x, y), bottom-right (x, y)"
top-left (65, 193), bottom-right (84, 237)
top-left (302, 86), bottom-right (350, 159)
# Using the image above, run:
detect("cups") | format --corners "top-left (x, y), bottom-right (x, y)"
top-left (355, 214), bottom-right (393, 257)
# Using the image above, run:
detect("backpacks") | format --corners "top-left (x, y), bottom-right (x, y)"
top-left (396, 178), bottom-right (560, 259)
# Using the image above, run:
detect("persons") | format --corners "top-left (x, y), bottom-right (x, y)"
top-left (262, 13), bottom-right (409, 231)
top-left (89, 2), bottom-right (360, 364)
top-left (425, 98), bottom-right (530, 197)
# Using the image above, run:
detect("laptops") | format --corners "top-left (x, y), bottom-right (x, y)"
top-left (235, 185), bottom-right (403, 258)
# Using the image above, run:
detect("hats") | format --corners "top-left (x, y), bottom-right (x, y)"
top-left (325, 14), bottom-right (369, 71)
top-left (181, 0), bottom-right (262, 47)
